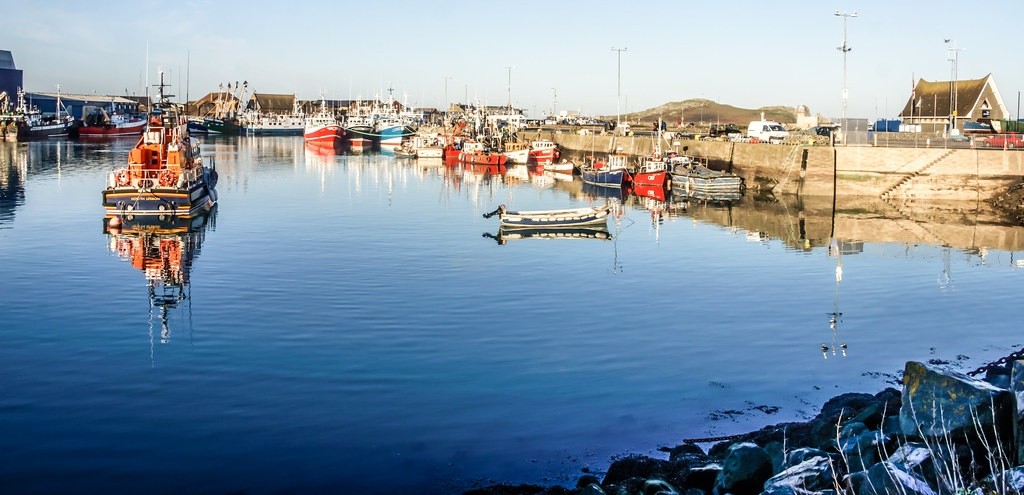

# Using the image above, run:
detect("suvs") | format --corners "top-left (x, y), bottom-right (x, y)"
top-left (710, 124), bottom-right (742, 138)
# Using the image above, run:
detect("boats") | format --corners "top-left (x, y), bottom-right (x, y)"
top-left (579, 117), bottom-right (743, 194)
top-left (483, 203), bottom-right (611, 227)
top-left (482, 223), bottom-right (613, 246)
top-left (103, 195), bottom-right (220, 365)
top-left (544, 159), bottom-right (574, 172)
top-left (581, 182), bottom-right (743, 244)
top-left (303, 140), bottom-right (553, 183)
top-left (101, 35), bottom-right (219, 208)
top-left (187, 80), bottom-right (305, 135)
top-left (16, 83), bottom-right (147, 138)
top-left (304, 66), bottom-right (559, 165)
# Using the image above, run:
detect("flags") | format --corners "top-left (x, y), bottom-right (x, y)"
top-left (912, 91), bottom-right (915, 100)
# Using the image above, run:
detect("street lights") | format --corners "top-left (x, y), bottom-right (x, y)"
top-left (610, 45), bottom-right (630, 124)
top-left (445, 76), bottom-right (454, 117)
top-left (833, 9), bottom-right (858, 118)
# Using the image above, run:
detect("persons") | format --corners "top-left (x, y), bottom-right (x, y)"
top-left (653, 120), bottom-right (659, 131)
top-left (662, 120), bottom-right (666, 132)
top-left (609, 120), bottom-right (615, 130)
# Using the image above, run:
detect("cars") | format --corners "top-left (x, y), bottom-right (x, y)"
top-left (984, 131), bottom-right (1024, 148)
top-left (809, 126), bottom-right (834, 136)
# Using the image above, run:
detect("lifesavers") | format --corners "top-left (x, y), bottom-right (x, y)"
top-left (486, 157), bottom-right (491, 162)
top-left (159, 170), bottom-right (174, 186)
top-left (115, 170), bottom-right (130, 186)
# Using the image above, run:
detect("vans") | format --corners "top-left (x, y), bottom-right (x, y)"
top-left (747, 120), bottom-right (789, 145)
